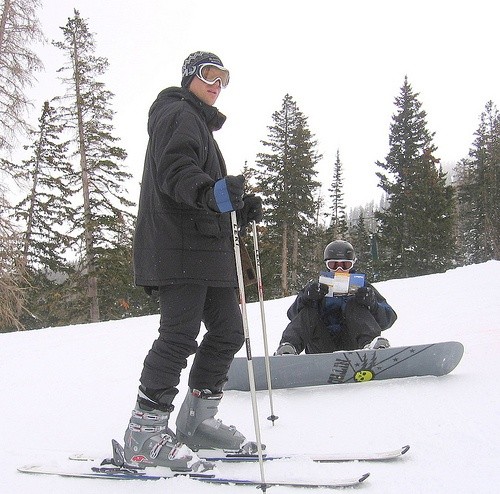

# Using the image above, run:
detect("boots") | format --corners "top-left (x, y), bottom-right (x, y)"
top-left (366, 336), bottom-right (391, 350)
top-left (120, 384), bottom-right (221, 479)
top-left (174, 386), bottom-right (266, 462)
top-left (272, 341), bottom-right (298, 355)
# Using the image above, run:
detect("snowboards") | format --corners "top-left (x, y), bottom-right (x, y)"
top-left (224, 341), bottom-right (465, 391)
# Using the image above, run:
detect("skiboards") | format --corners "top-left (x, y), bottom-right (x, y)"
top-left (15, 444), bottom-right (410, 489)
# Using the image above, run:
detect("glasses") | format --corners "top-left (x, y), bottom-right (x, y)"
top-left (196, 62), bottom-right (231, 89)
top-left (324, 259), bottom-right (356, 272)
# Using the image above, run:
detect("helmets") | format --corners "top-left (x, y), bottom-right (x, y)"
top-left (323, 239), bottom-right (356, 260)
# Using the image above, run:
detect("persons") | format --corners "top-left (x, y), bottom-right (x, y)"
top-left (124, 51), bottom-right (268, 474)
top-left (272, 239), bottom-right (399, 356)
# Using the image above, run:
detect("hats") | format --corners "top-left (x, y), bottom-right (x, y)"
top-left (180, 51), bottom-right (224, 88)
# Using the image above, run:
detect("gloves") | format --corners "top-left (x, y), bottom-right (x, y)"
top-left (354, 286), bottom-right (379, 312)
top-left (300, 278), bottom-right (330, 309)
top-left (236, 193), bottom-right (264, 227)
top-left (205, 174), bottom-right (245, 213)
